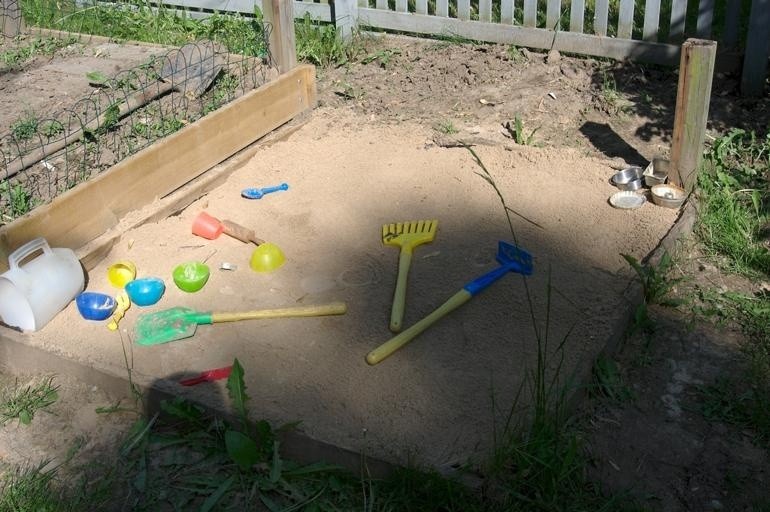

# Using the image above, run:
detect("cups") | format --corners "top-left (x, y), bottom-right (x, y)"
top-left (192, 211), bottom-right (223, 241)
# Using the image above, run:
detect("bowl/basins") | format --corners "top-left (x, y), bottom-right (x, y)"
top-left (610, 167), bottom-right (645, 191)
top-left (249, 242), bottom-right (286, 272)
top-left (650, 184), bottom-right (689, 208)
top-left (75, 261), bottom-right (209, 320)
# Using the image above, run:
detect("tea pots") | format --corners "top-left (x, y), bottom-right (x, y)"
top-left (0, 236), bottom-right (88, 333)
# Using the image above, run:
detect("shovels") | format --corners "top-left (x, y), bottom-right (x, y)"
top-left (134, 303), bottom-right (347, 347)
top-left (0, 42), bottom-right (225, 180)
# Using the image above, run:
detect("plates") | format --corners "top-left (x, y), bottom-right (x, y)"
top-left (609, 190), bottom-right (647, 210)
